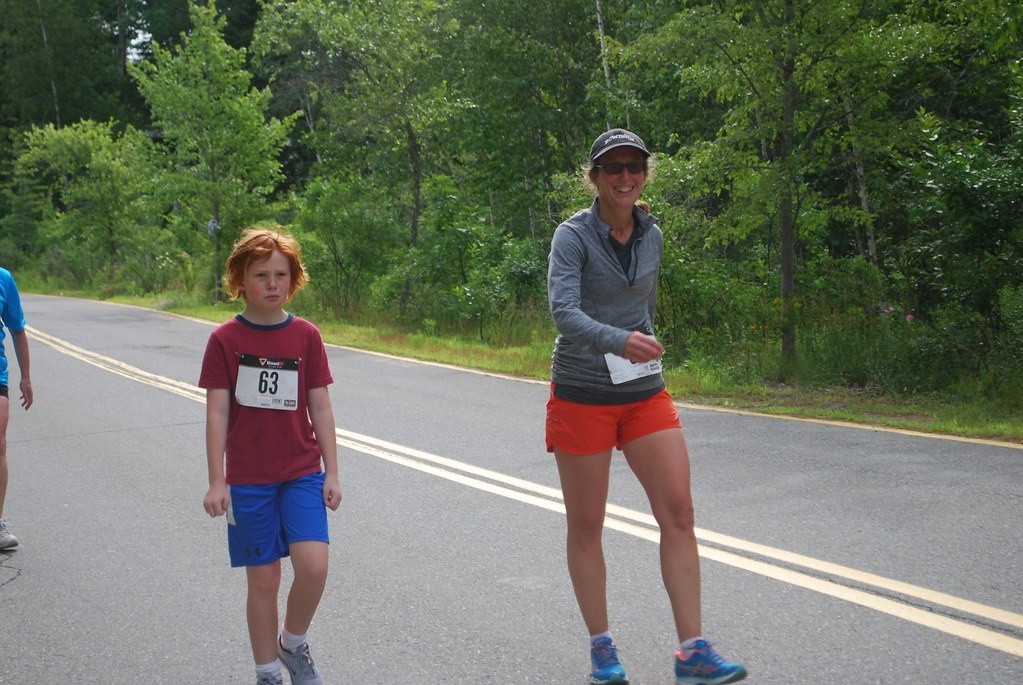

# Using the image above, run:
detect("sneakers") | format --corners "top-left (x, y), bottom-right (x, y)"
top-left (590, 636), bottom-right (629, 685)
top-left (256, 672), bottom-right (283, 685)
top-left (674, 639), bottom-right (747, 685)
top-left (0, 521), bottom-right (19, 548)
top-left (276, 634), bottom-right (324, 685)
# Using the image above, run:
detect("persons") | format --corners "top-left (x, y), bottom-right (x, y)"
top-left (0, 266), bottom-right (34, 548)
top-left (545, 128), bottom-right (747, 685)
top-left (199, 222), bottom-right (342, 685)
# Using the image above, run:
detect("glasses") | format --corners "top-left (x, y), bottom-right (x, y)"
top-left (591, 162), bottom-right (646, 175)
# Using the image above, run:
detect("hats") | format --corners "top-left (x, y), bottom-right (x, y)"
top-left (587, 129), bottom-right (652, 163)
